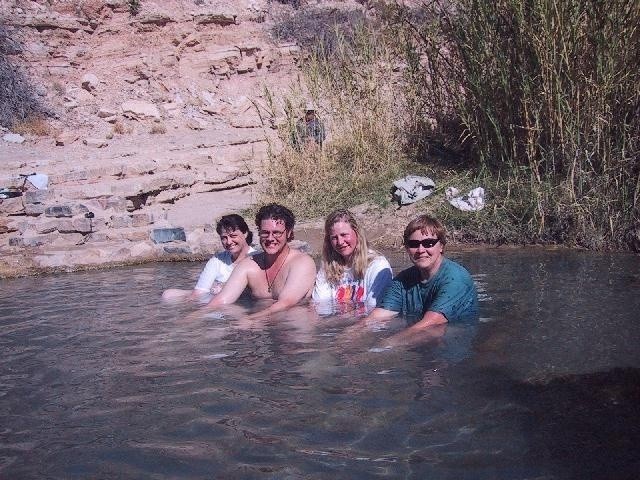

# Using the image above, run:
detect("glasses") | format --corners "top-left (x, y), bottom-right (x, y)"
top-left (259, 228), bottom-right (288, 239)
top-left (405, 238), bottom-right (440, 248)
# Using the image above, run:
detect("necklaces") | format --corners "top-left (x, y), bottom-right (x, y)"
top-left (263, 247), bottom-right (290, 293)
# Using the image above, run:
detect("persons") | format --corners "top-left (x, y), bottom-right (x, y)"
top-left (171, 203), bottom-right (317, 342)
top-left (289, 106), bottom-right (327, 156)
top-left (161, 213), bottom-right (258, 314)
top-left (328, 214), bottom-right (479, 373)
top-left (283, 208), bottom-right (393, 342)
